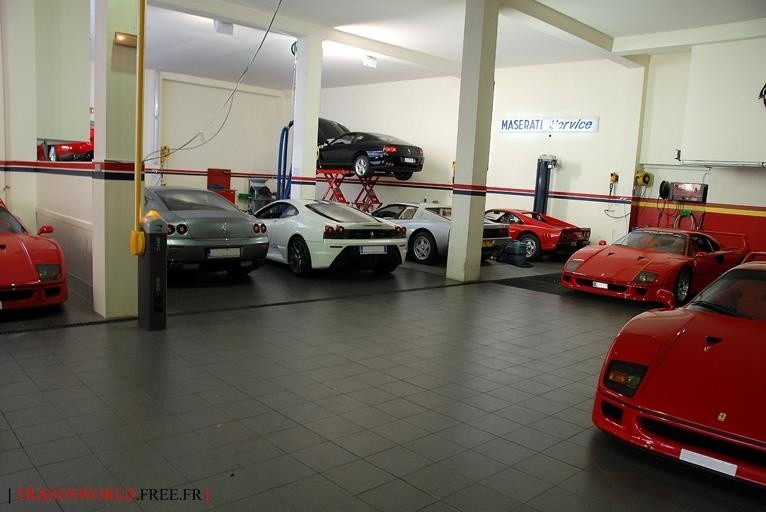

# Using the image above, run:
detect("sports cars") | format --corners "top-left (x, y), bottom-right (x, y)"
top-left (557, 224), bottom-right (739, 310)
top-left (591, 253), bottom-right (766, 495)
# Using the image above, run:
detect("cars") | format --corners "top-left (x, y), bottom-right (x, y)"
top-left (485, 207), bottom-right (591, 262)
top-left (461, 254), bottom-right (589, 300)
top-left (366, 202), bottom-right (509, 267)
top-left (245, 198), bottom-right (408, 286)
top-left (36, 123), bottom-right (94, 163)
top-left (0, 197), bottom-right (66, 321)
top-left (142, 182), bottom-right (269, 281)
top-left (316, 116), bottom-right (424, 179)
